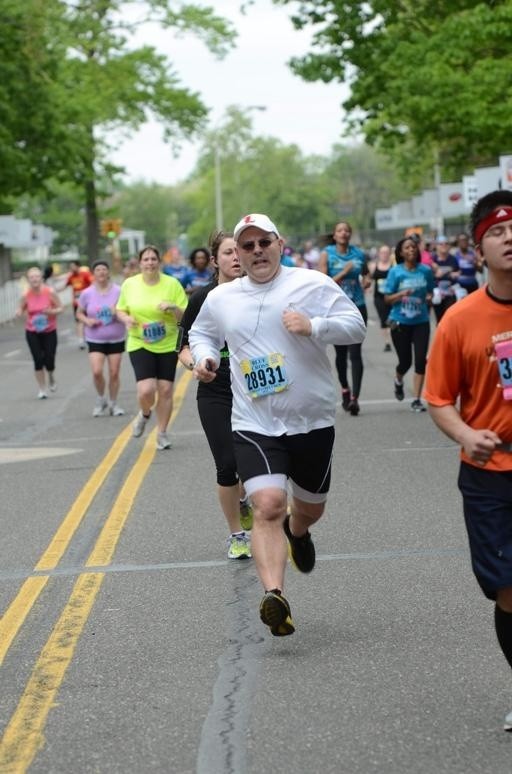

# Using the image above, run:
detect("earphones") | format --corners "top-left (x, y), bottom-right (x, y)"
top-left (219, 243), bottom-right (282, 359)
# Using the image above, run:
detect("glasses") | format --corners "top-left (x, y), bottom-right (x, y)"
top-left (476, 223), bottom-right (510, 240)
top-left (236, 239), bottom-right (279, 252)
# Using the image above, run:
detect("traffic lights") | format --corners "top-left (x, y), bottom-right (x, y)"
top-left (102, 221), bottom-right (120, 239)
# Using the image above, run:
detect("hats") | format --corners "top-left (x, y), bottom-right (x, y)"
top-left (234, 214), bottom-right (279, 238)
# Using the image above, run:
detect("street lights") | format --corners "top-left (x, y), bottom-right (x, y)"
top-left (384, 123), bottom-right (443, 239)
top-left (213, 103), bottom-right (267, 235)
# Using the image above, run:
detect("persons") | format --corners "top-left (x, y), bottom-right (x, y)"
top-left (318, 221), bottom-right (373, 419)
top-left (175, 229), bottom-right (255, 560)
top-left (179, 247), bottom-right (213, 297)
top-left (423, 189), bottom-right (511, 734)
top-left (384, 236), bottom-right (434, 413)
top-left (57, 262), bottom-right (95, 350)
top-left (116, 244), bottom-right (190, 451)
top-left (77, 260), bottom-right (124, 417)
top-left (427, 236), bottom-right (460, 326)
top-left (43, 266), bottom-right (56, 291)
top-left (410, 232), bottom-right (437, 272)
top-left (14, 266), bottom-right (65, 400)
top-left (163, 248), bottom-right (188, 289)
top-left (453, 231), bottom-right (483, 296)
top-left (280, 237), bottom-right (319, 270)
top-left (368, 245), bottom-right (394, 352)
top-left (189, 212), bottom-right (366, 636)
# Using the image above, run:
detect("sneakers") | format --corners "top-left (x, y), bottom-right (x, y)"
top-left (383, 344), bottom-right (391, 349)
top-left (38, 390), bottom-right (46, 397)
top-left (350, 400), bottom-right (360, 414)
top-left (395, 379), bottom-right (406, 401)
top-left (134, 411), bottom-right (147, 434)
top-left (51, 385), bottom-right (56, 394)
top-left (259, 589), bottom-right (295, 636)
top-left (158, 435), bottom-right (169, 446)
top-left (114, 406), bottom-right (125, 416)
top-left (411, 401), bottom-right (425, 411)
top-left (343, 390), bottom-right (350, 411)
top-left (239, 496), bottom-right (252, 530)
top-left (284, 514), bottom-right (316, 573)
top-left (93, 401), bottom-right (106, 415)
top-left (227, 532), bottom-right (253, 560)
